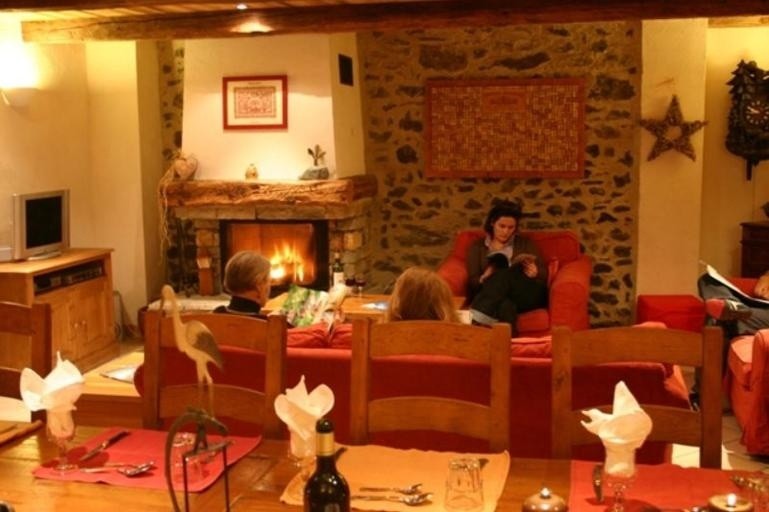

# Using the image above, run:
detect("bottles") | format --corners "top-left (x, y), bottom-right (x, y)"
top-left (305, 421), bottom-right (345, 511)
top-left (244, 163), bottom-right (258, 179)
top-left (331, 248), bottom-right (348, 285)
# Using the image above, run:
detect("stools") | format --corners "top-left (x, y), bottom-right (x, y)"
top-left (634, 292), bottom-right (706, 331)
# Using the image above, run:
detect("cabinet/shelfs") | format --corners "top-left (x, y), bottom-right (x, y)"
top-left (1, 246), bottom-right (121, 402)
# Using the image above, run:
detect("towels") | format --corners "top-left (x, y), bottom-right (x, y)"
top-left (17, 349), bottom-right (83, 435)
top-left (270, 373), bottom-right (337, 455)
top-left (581, 376), bottom-right (654, 476)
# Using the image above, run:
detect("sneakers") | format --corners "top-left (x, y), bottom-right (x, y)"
top-left (704, 298), bottom-right (753, 321)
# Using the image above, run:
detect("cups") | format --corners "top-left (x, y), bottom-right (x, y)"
top-left (456, 309), bottom-right (472, 324)
top-left (443, 458), bottom-right (484, 511)
top-left (172, 424), bottom-right (201, 484)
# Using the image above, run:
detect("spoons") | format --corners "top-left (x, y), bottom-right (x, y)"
top-left (353, 484), bottom-right (435, 505)
top-left (78, 465), bottom-right (154, 475)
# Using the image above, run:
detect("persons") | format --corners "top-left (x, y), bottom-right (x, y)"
top-left (462, 201), bottom-right (549, 337)
top-left (387, 267), bottom-right (459, 323)
top-left (215, 251), bottom-right (297, 330)
top-left (687, 259), bottom-right (769, 412)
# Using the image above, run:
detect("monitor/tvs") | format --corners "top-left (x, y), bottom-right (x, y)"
top-left (12, 189), bottom-right (69, 260)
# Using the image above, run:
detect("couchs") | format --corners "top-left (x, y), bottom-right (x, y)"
top-left (130, 322), bottom-right (693, 465)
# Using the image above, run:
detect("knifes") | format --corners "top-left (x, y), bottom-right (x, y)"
top-left (81, 429), bottom-right (131, 463)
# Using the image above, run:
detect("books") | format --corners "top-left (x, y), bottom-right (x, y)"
top-left (277, 281), bottom-right (351, 328)
top-left (487, 248), bottom-right (537, 266)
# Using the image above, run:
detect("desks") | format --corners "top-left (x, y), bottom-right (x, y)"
top-left (264, 286), bottom-right (466, 319)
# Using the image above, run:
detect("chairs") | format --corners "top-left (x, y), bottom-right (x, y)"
top-left (721, 269), bottom-right (769, 461)
top-left (548, 322), bottom-right (729, 471)
top-left (0, 298), bottom-right (58, 427)
top-left (442, 227), bottom-right (594, 330)
top-left (141, 307), bottom-right (289, 444)
top-left (348, 316), bottom-right (512, 458)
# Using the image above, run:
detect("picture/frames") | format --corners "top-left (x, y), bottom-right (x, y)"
top-left (421, 75), bottom-right (587, 182)
top-left (221, 74), bottom-right (289, 130)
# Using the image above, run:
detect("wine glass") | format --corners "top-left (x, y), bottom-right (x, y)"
top-left (283, 433), bottom-right (314, 497)
top-left (346, 272), bottom-right (368, 299)
top-left (602, 460), bottom-right (636, 511)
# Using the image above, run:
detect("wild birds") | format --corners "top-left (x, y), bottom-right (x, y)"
top-left (152, 284), bottom-right (224, 421)
